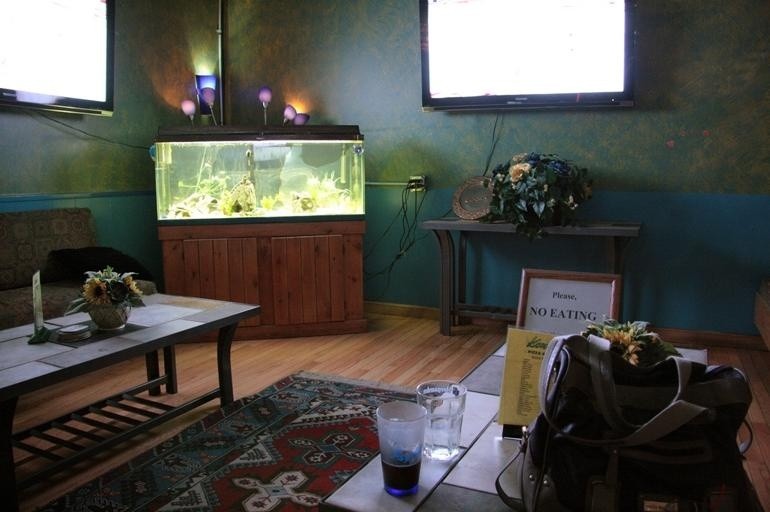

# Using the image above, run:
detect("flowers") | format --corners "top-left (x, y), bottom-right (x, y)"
top-left (580, 316), bottom-right (683, 365)
top-left (66, 266), bottom-right (147, 315)
top-left (476, 152), bottom-right (596, 245)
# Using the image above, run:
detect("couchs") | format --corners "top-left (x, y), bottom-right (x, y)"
top-left (0, 207), bottom-right (157, 329)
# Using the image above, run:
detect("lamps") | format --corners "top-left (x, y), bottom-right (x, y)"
top-left (181, 74), bottom-right (312, 128)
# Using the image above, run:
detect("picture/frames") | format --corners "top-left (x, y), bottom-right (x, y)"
top-left (516, 267), bottom-right (628, 334)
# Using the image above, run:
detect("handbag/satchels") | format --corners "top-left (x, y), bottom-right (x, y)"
top-left (520, 333), bottom-right (752, 511)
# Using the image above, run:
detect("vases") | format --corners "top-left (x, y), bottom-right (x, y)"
top-left (86, 302), bottom-right (131, 331)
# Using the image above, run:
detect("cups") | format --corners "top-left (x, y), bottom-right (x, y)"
top-left (416, 380), bottom-right (467, 461)
top-left (376, 400), bottom-right (428, 497)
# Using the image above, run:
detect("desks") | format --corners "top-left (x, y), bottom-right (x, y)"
top-left (421, 217), bottom-right (644, 337)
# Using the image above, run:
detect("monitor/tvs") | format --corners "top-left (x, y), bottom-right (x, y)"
top-left (418, 0), bottom-right (638, 114)
top-left (0, 0), bottom-right (118, 120)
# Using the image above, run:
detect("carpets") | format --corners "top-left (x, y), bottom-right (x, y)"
top-left (29, 370), bottom-right (417, 512)
